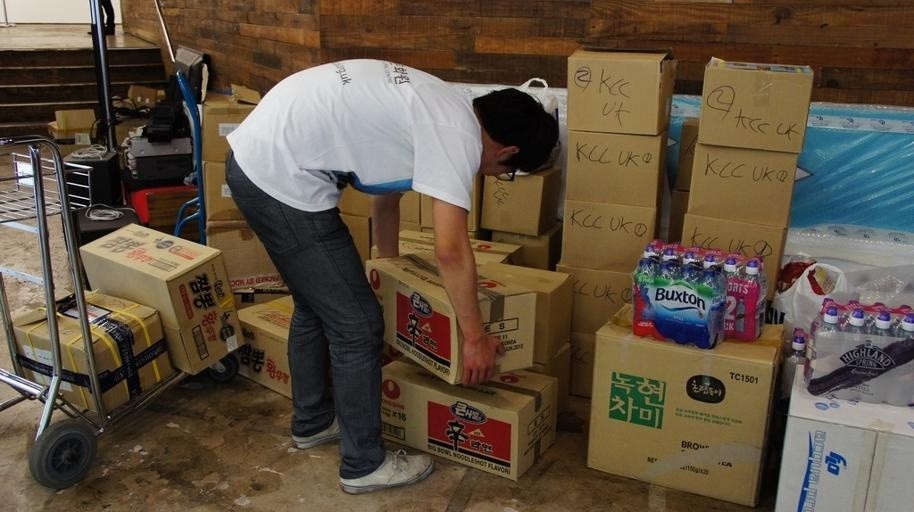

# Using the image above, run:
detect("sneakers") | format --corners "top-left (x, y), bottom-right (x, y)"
top-left (292, 412), bottom-right (343, 448)
top-left (338, 451), bottom-right (433, 494)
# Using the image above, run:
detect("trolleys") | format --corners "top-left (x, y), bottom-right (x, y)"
top-left (172, 68), bottom-right (290, 303)
top-left (0, 134), bottom-right (239, 491)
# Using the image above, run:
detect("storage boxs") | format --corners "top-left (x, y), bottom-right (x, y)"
top-left (12, 52), bottom-right (914, 512)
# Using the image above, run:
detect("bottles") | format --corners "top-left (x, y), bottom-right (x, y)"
top-left (632, 239), bottom-right (768, 348)
top-left (774, 293), bottom-right (913, 419)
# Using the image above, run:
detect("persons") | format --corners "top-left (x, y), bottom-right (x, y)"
top-left (224, 58), bottom-right (559, 495)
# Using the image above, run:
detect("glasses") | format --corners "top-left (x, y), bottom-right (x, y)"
top-left (495, 165), bottom-right (516, 182)
